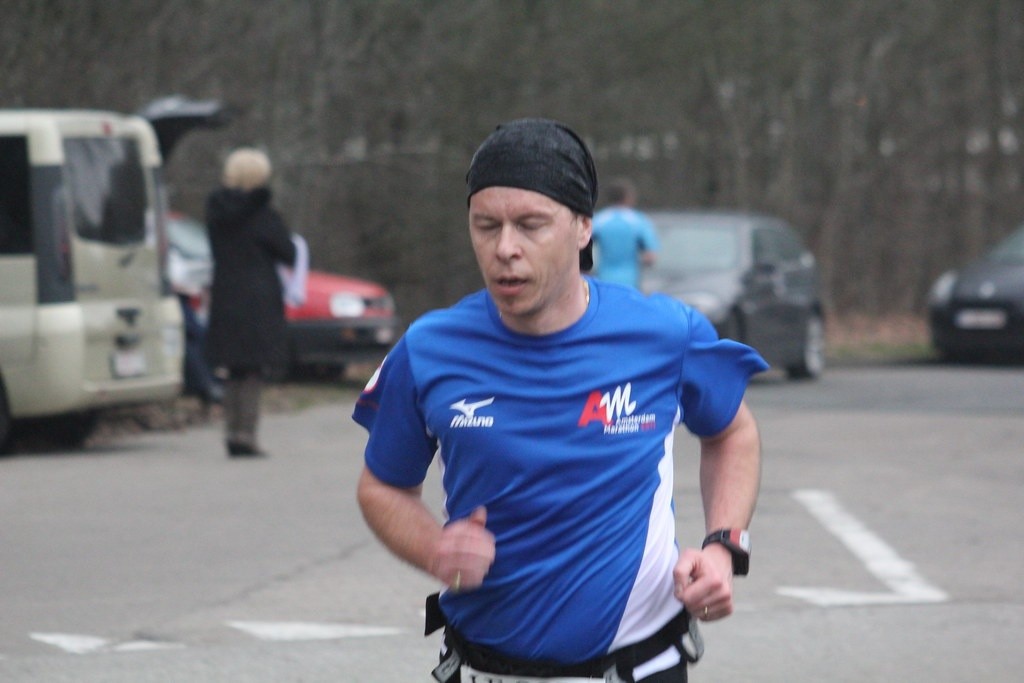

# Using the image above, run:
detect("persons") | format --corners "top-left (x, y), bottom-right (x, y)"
top-left (587, 180), bottom-right (660, 295)
top-left (350, 118), bottom-right (771, 683)
top-left (204, 148), bottom-right (298, 459)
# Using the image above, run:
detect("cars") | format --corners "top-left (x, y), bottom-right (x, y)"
top-left (922, 218), bottom-right (1024, 368)
top-left (635, 209), bottom-right (831, 387)
top-left (159, 206), bottom-right (401, 390)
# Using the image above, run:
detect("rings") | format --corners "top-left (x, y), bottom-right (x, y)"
top-left (704, 606), bottom-right (708, 619)
top-left (454, 571), bottom-right (461, 592)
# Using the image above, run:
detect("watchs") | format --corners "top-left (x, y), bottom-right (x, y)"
top-left (702, 528), bottom-right (752, 577)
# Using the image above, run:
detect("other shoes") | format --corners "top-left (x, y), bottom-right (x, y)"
top-left (225, 440), bottom-right (261, 456)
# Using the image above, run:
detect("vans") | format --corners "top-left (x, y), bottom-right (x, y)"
top-left (0, 108), bottom-right (188, 458)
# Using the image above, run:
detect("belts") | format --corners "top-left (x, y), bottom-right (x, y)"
top-left (440, 645), bottom-right (682, 683)
